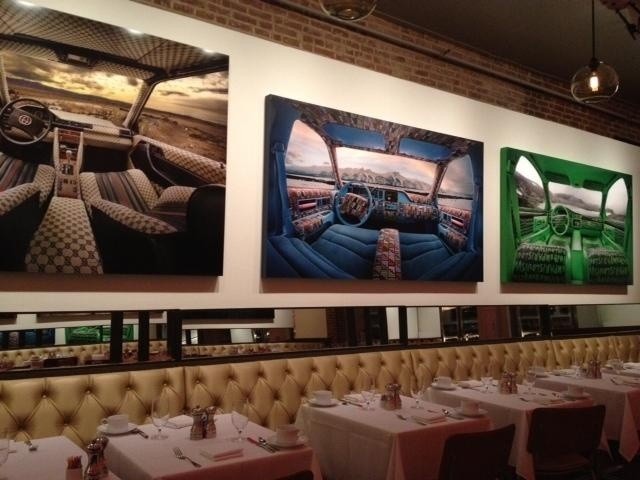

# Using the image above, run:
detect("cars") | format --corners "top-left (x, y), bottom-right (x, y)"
top-left (312, 390), bottom-right (332, 404)
top-left (276, 425), bottom-right (305, 442)
top-left (461, 399), bottom-right (482, 412)
top-left (65, 466), bottom-right (82, 480)
top-left (568, 385), bottom-right (585, 395)
top-left (100, 415), bottom-right (128, 432)
top-left (434, 376), bottom-right (451, 386)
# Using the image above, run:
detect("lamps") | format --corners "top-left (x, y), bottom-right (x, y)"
top-left (440, 425), bottom-right (517, 480)
top-left (0, 148), bottom-right (56, 271)
top-left (525, 404), bottom-right (610, 478)
top-left (513, 234), bottom-right (570, 283)
top-left (78, 167), bottom-right (226, 273)
top-left (268, 222), bottom-right (387, 279)
top-left (581, 234), bottom-right (630, 278)
top-left (389, 229), bottom-right (482, 282)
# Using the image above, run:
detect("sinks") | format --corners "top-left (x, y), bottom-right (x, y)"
top-left (0, 323), bottom-right (638, 449)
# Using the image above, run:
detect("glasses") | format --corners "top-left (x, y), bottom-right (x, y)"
top-left (572, 2), bottom-right (619, 103)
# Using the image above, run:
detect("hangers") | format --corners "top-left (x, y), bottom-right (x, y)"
top-left (259, 438), bottom-right (279, 450)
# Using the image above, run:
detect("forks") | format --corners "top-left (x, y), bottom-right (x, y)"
top-left (173, 447), bottom-right (201, 468)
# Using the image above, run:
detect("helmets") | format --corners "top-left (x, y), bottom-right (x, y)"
top-left (431, 383), bottom-right (456, 390)
top-left (270, 435), bottom-right (306, 448)
top-left (309, 398), bottom-right (339, 406)
top-left (457, 405), bottom-right (487, 417)
top-left (564, 394), bottom-right (589, 399)
top-left (95, 424), bottom-right (137, 434)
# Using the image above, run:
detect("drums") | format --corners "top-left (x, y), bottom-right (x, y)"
top-left (248, 437), bottom-right (273, 453)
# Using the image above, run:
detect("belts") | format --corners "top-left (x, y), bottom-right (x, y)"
top-left (362, 375), bottom-right (376, 417)
top-left (526, 372), bottom-right (535, 400)
top-left (480, 364), bottom-right (493, 396)
top-left (409, 376), bottom-right (426, 411)
top-left (231, 398), bottom-right (249, 442)
top-left (573, 351), bottom-right (585, 379)
top-left (151, 398), bottom-right (170, 440)
top-left (1, 437), bottom-right (8, 480)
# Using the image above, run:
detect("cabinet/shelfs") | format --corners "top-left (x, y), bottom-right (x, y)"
top-left (510, 373), bottom-right (518, 394)
top-left (588, 361), bottom-right (596, 378)
top-left (204, 405), bottom-right (218, 436)
top-left (385, 384), bottom-right (396, 410)
top-left (84, 444), bottom-right (102, 480)
top-left (596, 361), bottom-right (602, 379)
top-left (394, 383), bottom-right (401, 408)
top-left (500, 371), bottom-right (510, 393)
top-left (190, 406), bottom-right (204, 440)
top-left (92, 437), bottom-right (109, 480)
